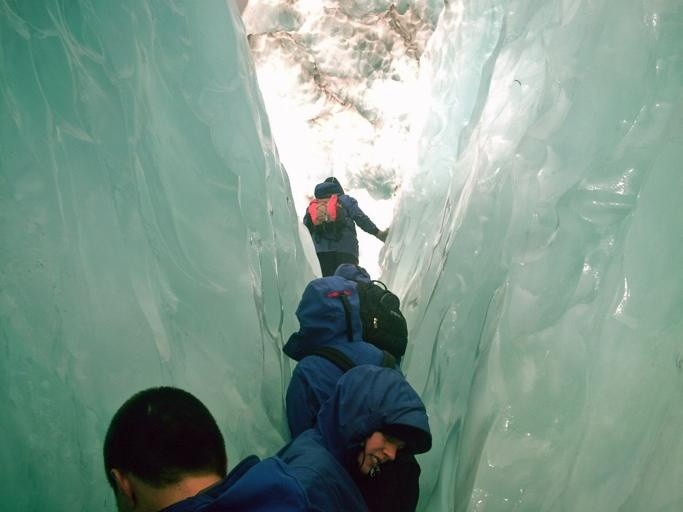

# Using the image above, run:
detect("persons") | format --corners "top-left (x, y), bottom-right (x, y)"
top-left (335, 262), bottom-right (401, 367)
top-left (301, 177), bottom-right (391, 279)
top-left (281, 363), bottom-right (432, 511)
top-left (285, 275), bottom-right (422, 511)
top-left (104, 384), bottom-right (310, 511)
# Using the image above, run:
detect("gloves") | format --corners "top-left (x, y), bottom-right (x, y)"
top-left (377, 228), bottom-right (388, 241)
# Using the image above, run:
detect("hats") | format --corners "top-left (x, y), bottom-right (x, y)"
top-left (392, 413), bottom-right (431, 456)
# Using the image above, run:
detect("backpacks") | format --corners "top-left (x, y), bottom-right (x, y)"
top-left (351, 278), bottom-right (408, 357)
top-left (305, 194), bottom-right (345, 241)
